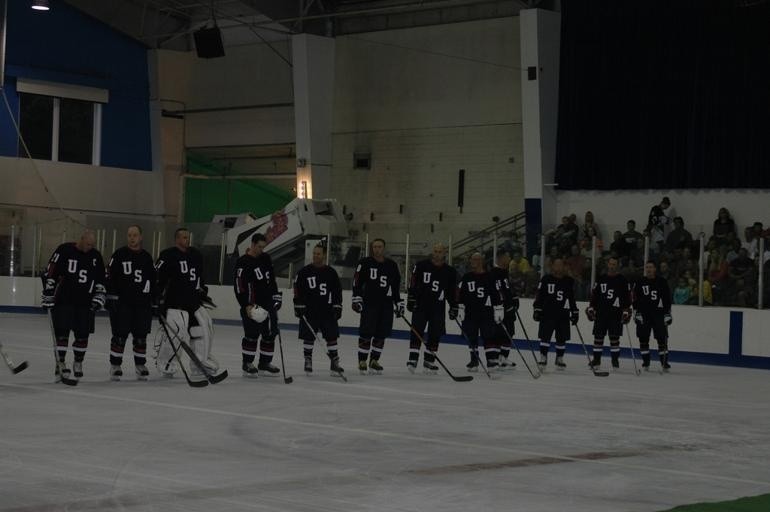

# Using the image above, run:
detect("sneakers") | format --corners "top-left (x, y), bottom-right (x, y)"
top-left (661, 362), bottom-right (671, 372)
top-left (368, 357), bottom-right (384, 375)
top-left (304, 360), bottom-right (312, 372)
top-left (73, 362), bottom-right (84, 377)
top-left (407, 359), bottom-right (417, 375)
top-left (487, 361), bottom-right (505, 373)
top-left (642, 361), bottom-right (651, 371)
top-left (330, 362), bottom-right (345, 372)
top-left (108, 364), bottom-right (122, 377)
top-left (611, 360), bottom-right (619, 371)
top-left (502, 356), bottom-right (518, 369)
top-left (588, 359), bottom-right (601, 370)
top-left (257, 362), bottom-right (280, 373)
top-left (554, 356), bottom-right (567, 369)
top-left (466, 361), bottom-right (480, 372)
top-left (358, 360), bottom-right (369, 375)
top-left (538, 353), bottom-right (548, 369)
top-left (54, 363), bottom-right (70, 375)
top-left (242, 362), bottom-right (258, 374)
top-left (498, 354), bottom-right (507, 371)
top-left (134, 364), bottom-right (149, 376)
top-left (423, 360), bottom-right (439, 375)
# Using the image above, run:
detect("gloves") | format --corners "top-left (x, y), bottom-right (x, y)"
top-left (351, 295), bottom-right (363, 312)
top-left (407, 297), bottom-right (418, 313)
top-left (271, 292), bottom-right (282, 310)
top-left (293, 297), bottom-right (311, 321)
top-left (449, 304), bottom-right (459, 320)
top-left (88, 282), bottom-right (108, 313)
top-left (494, 296), bottom-right (672, 328)
top-left (40, 278), bottom-right (57, 311)
top-left (394, 298), bottom-right (406, 318)
top-left (152, 292), bottom-right (165, 317)
top-left (330, 303), bottom-right (342, 320)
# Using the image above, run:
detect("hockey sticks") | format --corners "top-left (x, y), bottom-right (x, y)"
top-left (274, 309), bottom-right (293, 384)
top-left (0, 346), bottom-right (28, 375)
top-left (40, 269), bottom-right (80, 386)
top-left (625, 322), bottom-right (669, 377)
top-left (158, 314), bottom-right (228, 387)
top-left (571, 316), bottom-right (610, 376)
top-left (392, 307), bottom-right (491, 381)
top-left (500, 307), bottom-right (543, 379)
top-left (302, 315), bottom-right (347, 381)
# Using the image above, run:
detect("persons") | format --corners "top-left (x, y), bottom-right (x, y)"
top-left (42, 228), bottom-right (107, 383)
top-left (633, 260), bottom-right (672, 372)
top-left (233, 233), bottom-right (283, 377)
top-left (293, 244), bottom-right (344, 376)
top-left (585, 257), bottom-right (632, 370)
top-left (455, 195), bottom-right (770, 309)
top-left (104, 224), bottom-right (160, 380)
top-left (533, 259), bottom-right (579, 369)
top-left (352, 238), bottom-right (405, 376)
top-left (460, 251), bottom-right (520, 369)
top-left (153, 228), bottom-right (223, 383)
top-left (406, 241), bottom-right (459, 376)
